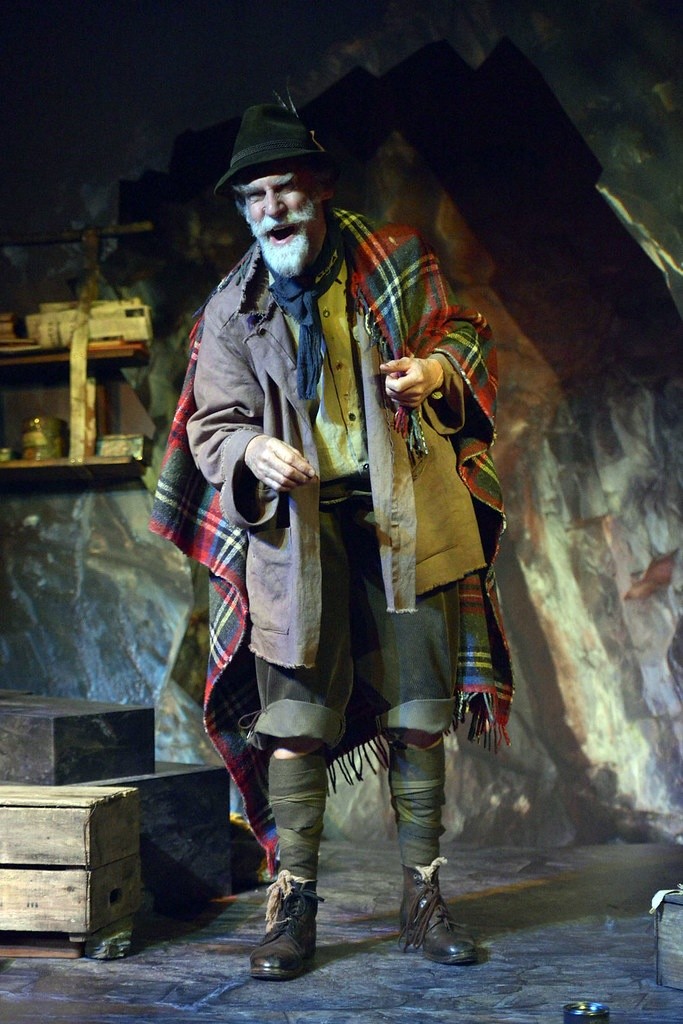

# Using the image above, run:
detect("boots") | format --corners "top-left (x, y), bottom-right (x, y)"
top-left (249, 871), bottom-right (325, 979)
top-left (397, 857), bottom-right (476, 962)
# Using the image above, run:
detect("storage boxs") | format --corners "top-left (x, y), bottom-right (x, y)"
top-left (0, 690), bottom-right (234, 932)
top-left (656, 892), bottom-right (683, 990)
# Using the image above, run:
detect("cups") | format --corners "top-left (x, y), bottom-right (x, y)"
top-left (563, 1001), bottom-right (610, 1024)
top-left (21, 417), bottom-right (68, 461)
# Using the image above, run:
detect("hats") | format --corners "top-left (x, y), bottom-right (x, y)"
top-left (214, 102), bottom-right (342, 200)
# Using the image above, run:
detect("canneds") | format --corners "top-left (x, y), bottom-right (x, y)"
top-left (20, 415), bottom-right (67, 462)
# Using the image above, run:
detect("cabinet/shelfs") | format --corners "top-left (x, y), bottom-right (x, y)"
top-left (0, 222), bottom-right (156, 490)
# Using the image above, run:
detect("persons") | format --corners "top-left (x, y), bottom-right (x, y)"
top-left (149, 102), bottom-right (516, 980)
